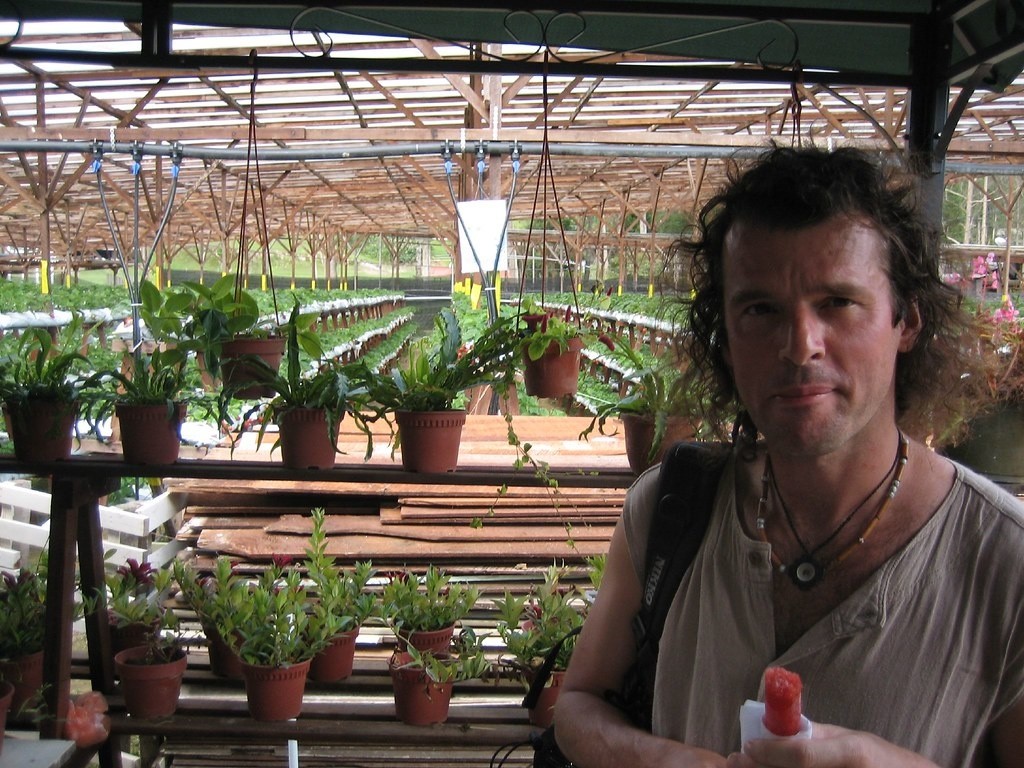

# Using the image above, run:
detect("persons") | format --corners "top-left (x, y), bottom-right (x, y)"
top-left (553, 142), bottom-right (1024, 768)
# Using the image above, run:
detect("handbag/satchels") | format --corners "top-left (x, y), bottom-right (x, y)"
top-left (489, 437), bottom-right (733, 768)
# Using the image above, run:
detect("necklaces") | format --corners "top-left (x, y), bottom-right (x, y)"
top-left (770, 428), bottom-right (902, 585)
top-left (756, 435), bottom-right (909, 577)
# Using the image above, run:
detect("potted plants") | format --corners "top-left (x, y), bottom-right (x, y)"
top-left (0, 272), bottom-right (729, 758)
